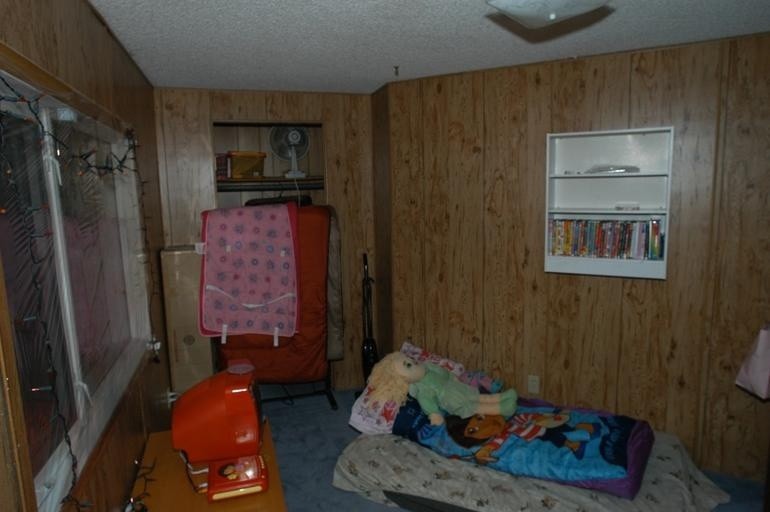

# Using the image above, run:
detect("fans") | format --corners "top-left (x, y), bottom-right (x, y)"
top-left (268, 125), bottom-right (309, 178)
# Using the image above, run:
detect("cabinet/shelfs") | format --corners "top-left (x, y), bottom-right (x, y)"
top-left (544, 125), bottom-right (673, 280)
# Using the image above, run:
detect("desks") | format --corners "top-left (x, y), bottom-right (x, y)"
top-left (129, 413), bottom-right (288, 512)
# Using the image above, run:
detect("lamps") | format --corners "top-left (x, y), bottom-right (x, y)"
top-left (485, 0), bottom-right (610, 29)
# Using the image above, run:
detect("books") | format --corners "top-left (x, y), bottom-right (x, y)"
top-left (548, 217), bottom-right (665, 261)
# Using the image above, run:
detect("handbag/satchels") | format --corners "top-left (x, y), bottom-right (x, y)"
top-left (735, 328), bottom-right (770, 399)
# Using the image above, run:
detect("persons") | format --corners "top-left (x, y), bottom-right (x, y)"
top-left (368, 351), bottom-right (517, 426)
top-left (218, 459), bottom-right (257, 480)
top-left (446, 415), bottom-right (601, 466)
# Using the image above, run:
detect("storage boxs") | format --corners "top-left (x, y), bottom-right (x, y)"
top-left (227, 150), bottom-right (267, 179)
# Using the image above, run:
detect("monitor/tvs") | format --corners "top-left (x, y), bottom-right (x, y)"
top-left (172, 358), bottom-right (261, 462)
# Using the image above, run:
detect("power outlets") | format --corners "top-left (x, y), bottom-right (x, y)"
top-left (528, 376), bottom-right (539, 394)
